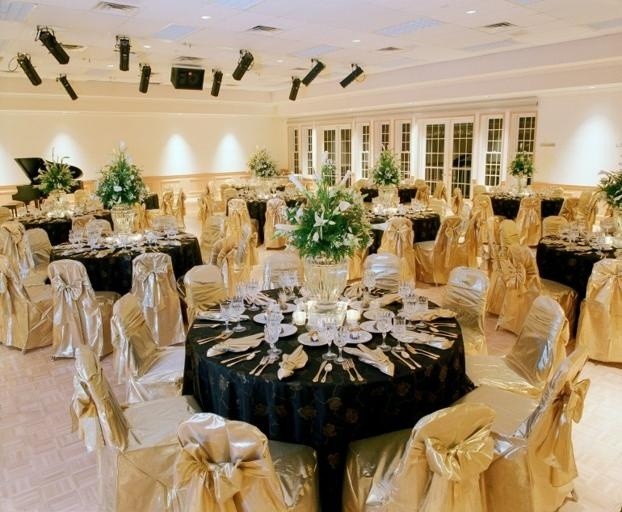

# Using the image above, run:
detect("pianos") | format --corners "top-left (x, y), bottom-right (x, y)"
top-left (13, 157), bottom-right (83, 209)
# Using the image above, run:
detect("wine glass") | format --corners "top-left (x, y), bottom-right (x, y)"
top-left (369, 177), bottom-right (554, 220)
top-left (558, 217), bottom-right (617, 257)
top-left (218, 268), bottom-right (430, 365)
top-left (26, 198), bottom-right (96, 222)
top-left (66, 212), bottom-right (181, 251)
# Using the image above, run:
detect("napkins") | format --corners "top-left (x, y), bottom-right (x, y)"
top-left (342, 343), bottom-right (394, 377)
top-left (389, 329), bottom-right (454, 350)
top-left (278, 344), bottom-right (309, 380)
top-left (206, 338), bottom-right (261, 357)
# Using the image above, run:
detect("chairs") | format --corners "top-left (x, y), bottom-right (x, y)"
top-left (573, 257), bottom-right (620, 367)
top-left (1, 229), bottom-right (55, 352)
top-left (172, 413), bottom-right (317, 512)
top-left (440, 265), bottom-right (490, 356)
top-left (453, 340), bottom-right (591, 511)
top-left (47, 259), bottom-right (119, 361)
top-left (461, 295), bottom-right (571, 403)
top-left (343, 400), bottom-right (497, 511)
top-left (109, 294), bottom-right (186, 407)
top-left (67, 346), bottom-right (203, 510)
top-left (129, 251), bottom-right (187, 348)
top-left (2, 172), bottom-right (621, 329)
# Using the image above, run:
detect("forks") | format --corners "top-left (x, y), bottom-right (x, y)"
top-left (248, 356), bottom-right (277, 376)
top-left (193, 329), bottom-right (234, 345)
top-left (340, 359), bottom-right (364, 382)
top-left (405, 342), bottom-right (442, 360)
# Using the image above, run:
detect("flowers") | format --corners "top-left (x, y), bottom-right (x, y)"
top-left (89, 138), bottom-right (151, 211)
top-left (247, 144), bottom-right (278, 176)
top-left (367, 141), bottom-right (404, 187)
top-left (272, 146), bottom-right (375, 264)
top-left (29, 146), bottom-right (83, 195)
top-left (505, 150), bottom-right (535, 177)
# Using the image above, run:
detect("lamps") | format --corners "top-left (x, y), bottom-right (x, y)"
top-left (232, 48), bottom-right (255, 82)
top-left (209, 69), bottom-right (224, 97)
top-left (57, 75), bottom-right (78, 101)
top-left (288, 75), bottom-right (302, 100)
top-left (115, 36), bottom-right (131, 71)
top-left (338, 61), bottom-right (364, 89)
top-left (8, 52), bottom-right (41, 87)
top-left (301, 58), bottom-right (326, 88)
top-left (34, 24), bottom-right (69, 65)
top-left (138, 62), bottom-right (151, 94)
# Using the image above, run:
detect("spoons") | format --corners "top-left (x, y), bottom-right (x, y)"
top-left (321, 363), bottom-right (331, 383)
top-left (400, 350), bottom-right (423, 369)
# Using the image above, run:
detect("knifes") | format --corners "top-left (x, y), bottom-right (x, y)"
top-left (389, 349), bottom-right (416, 371)
top-left (312, 359), bottom-right (328, 383)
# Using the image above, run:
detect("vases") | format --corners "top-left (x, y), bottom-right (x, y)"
top-left (304, 256), bottom-right (350, 310)
